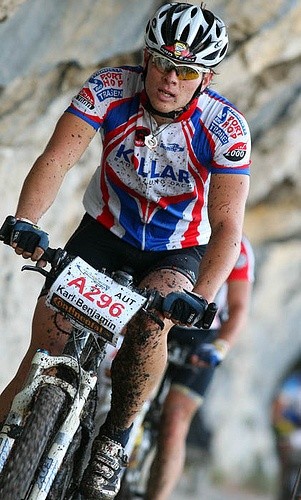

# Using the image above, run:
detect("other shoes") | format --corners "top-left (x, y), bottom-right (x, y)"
top-left (80, 434), bottom-right (129, 500)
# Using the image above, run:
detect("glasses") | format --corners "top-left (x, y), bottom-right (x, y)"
top-left (146, 46), bottom-right (211, 82)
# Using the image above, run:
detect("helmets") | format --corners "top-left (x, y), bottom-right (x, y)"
top-left (144, 2), bottom-right (229, 69)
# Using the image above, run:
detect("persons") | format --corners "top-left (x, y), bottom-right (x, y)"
top-left (271, 354), bottom-right (301, 500)
top-left (0, 2), bottom-right (250, 500)
top-left (97, 235), bottom-right (256, 500)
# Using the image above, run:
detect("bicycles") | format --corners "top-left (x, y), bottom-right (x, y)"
top-left (0, 215), bottom-right (219, 500)
top-left (276, 418), bottom-right (301, 500)
top-left (82, 334), bottom-right (224, 500)
